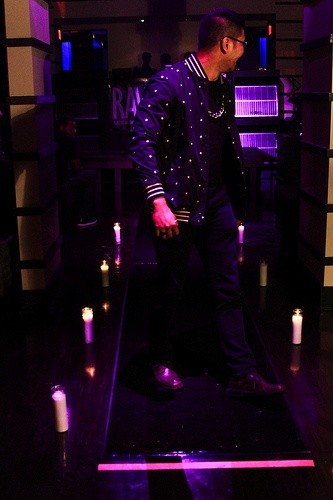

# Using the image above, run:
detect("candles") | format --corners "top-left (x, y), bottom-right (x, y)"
top-left (238, 223), bottom-right (245, 243)
top-left (114, 223), bottom-right (121, 242)
top-left (82, 307), bottom-right (93, 343)
top-left (100, 260), bottom-right (109, 287)
top-left (259, 261), bottom-right (267, 286)
top-left (292, 309), bottom-right (303, 344)
top-left (52, 386), bottom-right (68, 432)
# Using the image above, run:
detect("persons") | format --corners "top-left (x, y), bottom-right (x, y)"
top-left (53, 116), bottom-right (81, 172)
top-left (128, 8), bottom-right (289, 397)
top-left (160, 53), bottom-right (173, 66)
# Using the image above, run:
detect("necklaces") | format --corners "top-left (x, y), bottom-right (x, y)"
top-left (208, 94), bottom-right (225, 119)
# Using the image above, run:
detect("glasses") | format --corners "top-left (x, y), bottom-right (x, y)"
top-left (227, 36), bottom-right (248, 48)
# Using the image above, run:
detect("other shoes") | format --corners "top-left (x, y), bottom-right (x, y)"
top-left (224, 371), bottom-right (285, 398)
top-left (147, 365), bottom-right (185, 391)
